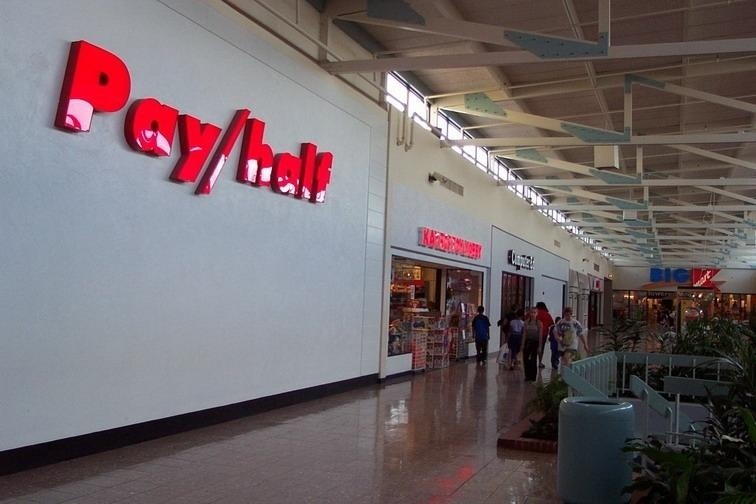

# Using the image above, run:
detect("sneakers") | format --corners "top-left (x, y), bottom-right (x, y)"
top-left (476, 356), bottom-right (487, 361)
top-left (551, 364), bottom-right (558, 369)
top-left (525, 377), bottom-right (536, 381)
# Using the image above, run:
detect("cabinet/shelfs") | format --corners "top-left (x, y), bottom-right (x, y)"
top-left (426, 327), bottom-right (449, 368)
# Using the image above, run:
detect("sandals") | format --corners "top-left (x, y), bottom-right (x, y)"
top-left (539, 363), bottom-right (545, 368)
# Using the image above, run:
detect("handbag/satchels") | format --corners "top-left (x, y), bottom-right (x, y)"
top-left (562, 331), bottom-right (574, 346)
top-left (496, 343), bottom-right (509, 364)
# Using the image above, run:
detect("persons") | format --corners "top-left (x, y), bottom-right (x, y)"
top-left (501, 302), bottom-right (588, 381)
top-left (472, 306), bottom-right (490, 362)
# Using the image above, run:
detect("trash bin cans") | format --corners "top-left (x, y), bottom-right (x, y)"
top-left (591, 324), bottom-right (609, 355)
top-left (557, 396), bottom-right (634, 504)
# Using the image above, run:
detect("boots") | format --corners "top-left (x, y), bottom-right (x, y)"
top-left (510, 358), bottom-right (519, 370)
